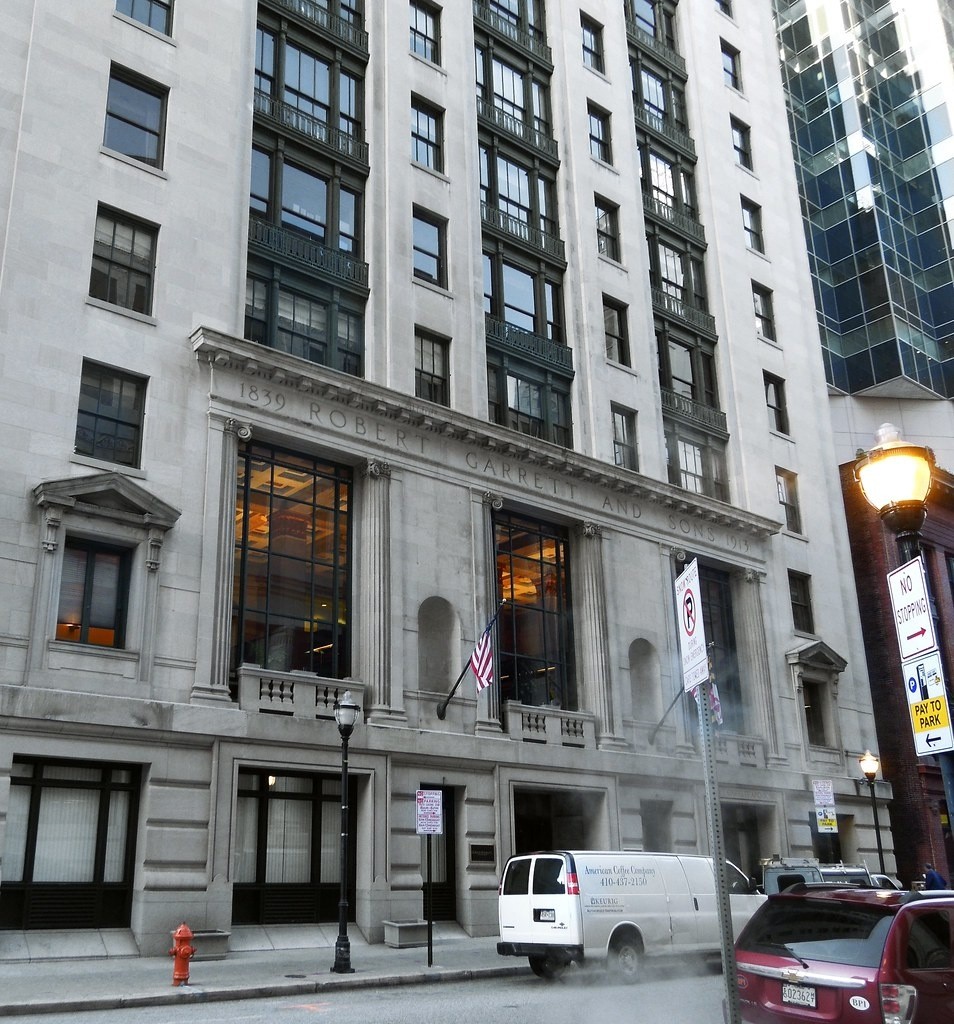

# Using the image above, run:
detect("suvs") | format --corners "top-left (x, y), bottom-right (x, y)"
top-left (722, 882), bottom-right (954, 1024)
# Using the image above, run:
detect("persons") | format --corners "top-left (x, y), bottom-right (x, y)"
top-left (924, 862), bottom-right (948, 891)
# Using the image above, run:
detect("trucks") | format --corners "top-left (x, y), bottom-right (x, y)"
top-left (496, 850), bottom-right (768, 984)
top-left (763, 854), bottom-right (900, 896)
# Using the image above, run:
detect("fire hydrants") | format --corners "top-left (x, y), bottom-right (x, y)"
top-left (168, 921), bottom-right (197, 985)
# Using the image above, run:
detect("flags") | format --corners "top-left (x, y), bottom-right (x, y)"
top-left (470, 625), bottom-right (493, 693)
top-left (689, 651), bottom-right (724, 725)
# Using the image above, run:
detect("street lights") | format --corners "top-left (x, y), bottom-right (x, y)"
top-left (330, 690), bottom-right (362, 974)
top-left (859, 749), bottom-right (887, 887)
top-left (852, 423), bottom-right (954, 840)
